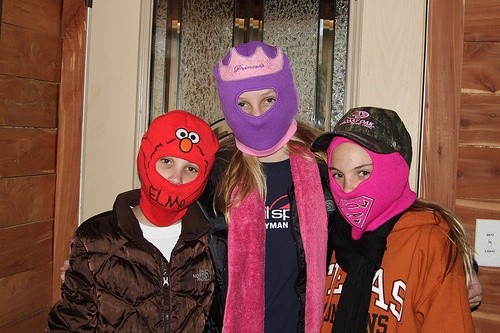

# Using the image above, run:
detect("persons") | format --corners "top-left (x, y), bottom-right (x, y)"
top-left (47, 109), bottom-right (218, 333)
top-left (59, 40), bottom-right (483, 308)
top-left (310, 107), bottom-right (475, 333)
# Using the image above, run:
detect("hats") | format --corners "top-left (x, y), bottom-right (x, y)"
top-left (310, 107), bottom-right (412, 169)
